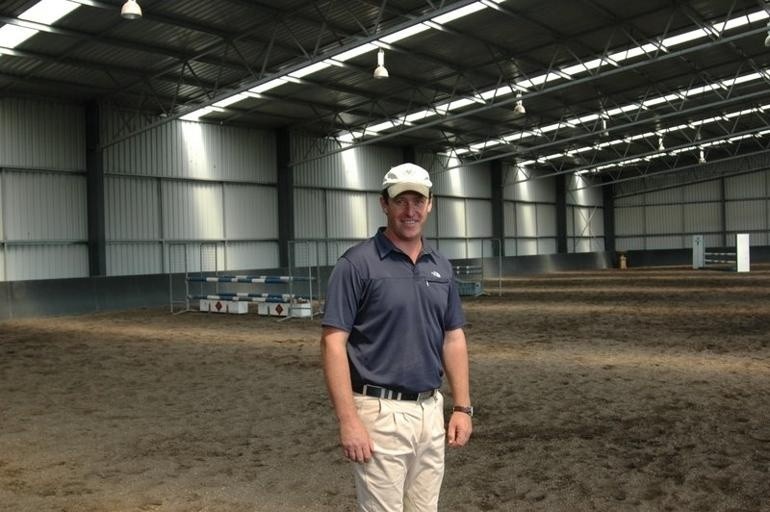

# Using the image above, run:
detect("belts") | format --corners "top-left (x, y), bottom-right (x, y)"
top-left (352, 382), bottom-right (434, 402)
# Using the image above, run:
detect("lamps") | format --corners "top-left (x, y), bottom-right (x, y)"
top-left (120, 0), bottom-right (143, 20)
top-left (513, 93), bottom-right (526, 115)
top-left (373, 50), bottom-right (389, 79)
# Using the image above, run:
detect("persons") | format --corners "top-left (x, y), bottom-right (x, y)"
top-left (320, 162), bottom-right (474, 512)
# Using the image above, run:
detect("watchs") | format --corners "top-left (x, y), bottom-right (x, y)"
top-left (452, 406), bottom-right (474, 416)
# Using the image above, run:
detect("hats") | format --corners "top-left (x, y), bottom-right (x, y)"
top-left (383, 162), bottom-right (433, 198)
